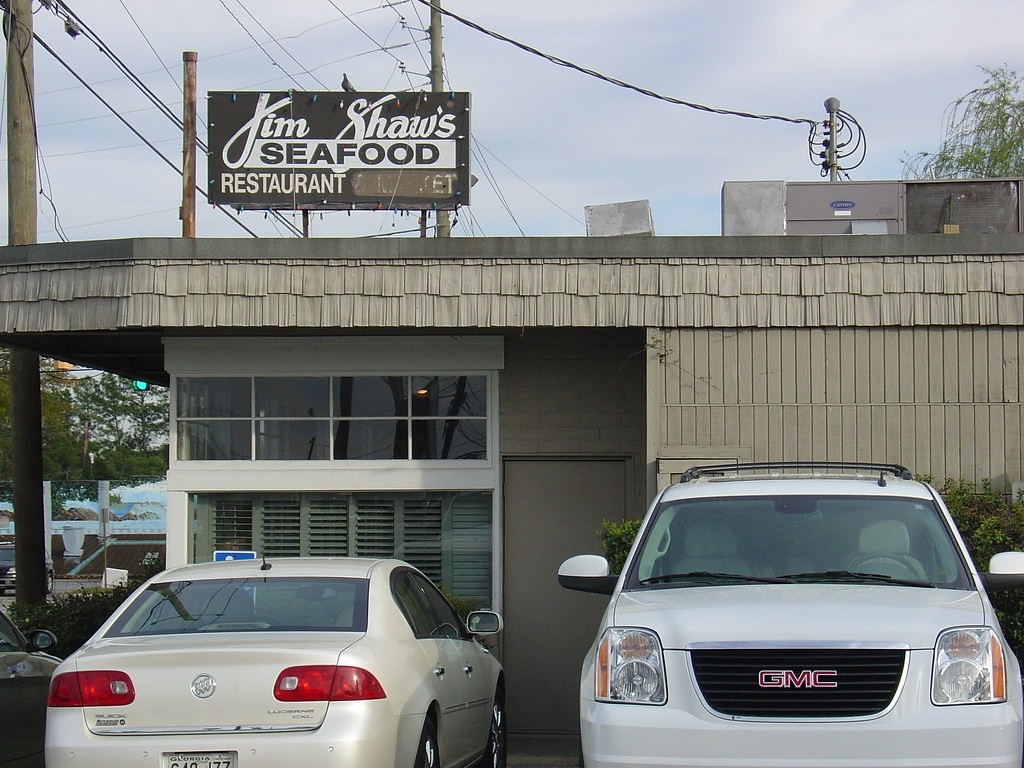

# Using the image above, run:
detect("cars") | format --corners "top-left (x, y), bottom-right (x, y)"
top-left (43, 558), bottom-right (509, 768)
top-left (0, 609), bottom-right (65, 767)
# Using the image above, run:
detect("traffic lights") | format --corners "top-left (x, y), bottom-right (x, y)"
top-left (133, 379), bottom-right (150, 391)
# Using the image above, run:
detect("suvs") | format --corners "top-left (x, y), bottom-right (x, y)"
top-left (558, 459), bottom-right (1024, 767)
top-left (0, 543), bottom-right (55, 596)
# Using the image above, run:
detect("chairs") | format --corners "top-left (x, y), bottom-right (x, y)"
top-left (672, 521), bottom-right (754, 578)
top-left (200, 589), bottom-right (254, 626)
top-left (849, 520), bottom-right (927, 582)
